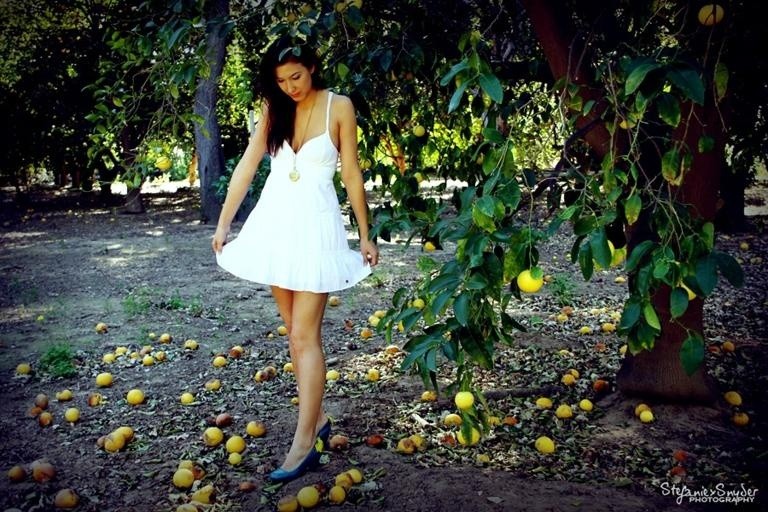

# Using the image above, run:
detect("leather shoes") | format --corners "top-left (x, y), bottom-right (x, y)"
top-left (270, 445), bottom-right (321, 483)
top-left (316, 419), bottom-right (332, 450)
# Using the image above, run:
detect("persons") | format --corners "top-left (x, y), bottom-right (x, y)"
top-left (213, 33), bottom-right (379, 481)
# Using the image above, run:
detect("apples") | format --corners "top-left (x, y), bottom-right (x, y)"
top-left (53, 488), bottom-right (78, 507)
top-left (348, 470), bottom-right (365, 484)
top-left (411, 434), bottom-right (425, 451)
top-left (180, 340), bottom-right (196, 355)
top-left (204, 378), bottom-right (221, 391)
top-left (536, 397), bottom-right (551, 412)
top-left (16, 362), bottom-right (31, 379)
top-left (85, 394), bottom-right (102, 406)
top-left (159, 333), bottom-right (171, 349)
top-left (179, 458), bottom-right (195, 472)
top-left (670, 466), bottom-right (685, 479)
top-left (149, 332), bottom-right (155, 343)
top-left (359, 158), bottom-right (371, 169)
top-left (297, 485), bottom-right (320, 507)
top-left (444, 412), bottom-right (462, 425)
top-left (266, 363), bottom-right (277, 377)
top-left (454, 391), bottom-right (474, 408)
top-left (284, 361), bottom-right (296, 373)
top-left (455, 426), bottom-right (480, 446)
top-left (732, 412), bottom-right (748, 424)
top-left (697, 6), bottom-right (724, 25)
top-left (33, 463), bottom-right (51, 482)
top-left (422, 386), bottom-right (443, 401)
top-left (614, 273), bottom-right (626, 286)
top-left (399, 437), bottom-right (413, 455)
top-left (387, 71), bottom-right (399, 82)
top-left (594, 379), bottom-right (608, 393)
top-left (277, 327), bottom-right (290, 336)
top-left (287, 13), bottom-right (297, 22)
top-left (413, 126), bottom-right (427, 137)
top-left (560, 372), bottom-right (576, 386)
top-left (156, 352), bottom-right (166, 361)
top-left (113, 341), bottom-right (127, 357)
top-left (519, 271), bottom-right (542, 293)
top-left (226, 453), bottom-right (243, 463)
top-left (95, 371), bottom-right (113, 388)
top-left (337, 2), bottom-right (348, 14)
top-left (129, 348), bottom-right (139, 360)
top-left (35, 315), bottom-right (47, 323)
top-left (252, 370), bottom-right (263, 383)
top-left (329, 296), bottom-right (340, 306)
top-left (101, 354), bottom-right (116, 366)
top-left (231, 344), bottom-right (244, 361)
top-left (178, 394), bottom-right (195, 404)
top-left (369, 366), bottom-right (380, 383)
top-left (579, 399), bottom-right (594, 411)
top-left (212, 356), bottom-right (226, 366)
top-left (600, 322), bottom-right (616, 334)
top-left (568, 367), bottom-right (580, 380)
top-left (328, 433), bottom-right (345, 449)
top-left (346, 0), bottom-right (363, 9)
top-left (423, 241), bottom-right (438, 254)
top-left (709, 345), bottom-right (720, 354)
top-left (405, 74), bottom-right (415, 81)
top-left (202, 425), bottom-right (223, 445)
top-left (554, 405), bottom-right (573, 420)
top-left (138, 343), bottom-right (151, 353)
top-left (95, 322), bottom-right (108, 337)
top-left (190, 487), bottom-right (216, 505)
top-left (302, 5), bottom-right (313, 14)
top-left (360, 307), bottom-right (410, 343)
top-left (172, 470), bottom-right (195, 491)
top-left (618, 344), bottom-right (630, 357)
top-left (65, 405), bottom-right (79, 421)
top-left (33, 394), bottom-right (48, 406)
top-left (723, 390), bottom-right (742, 405)
top-left (224, 436), bottom-right (248, 448)
top-left (578, 325), bottom-right (593, 335)
top-left (412, 298), bottom-right (426, 311)
top-left (741, 240), bottom-right (749, 252)
top-left (722, 340), bottom-right (735, 352)
top-left (414, 171), bottom-right (422, 183)
top-left (177, 503), bottom-right (198, 512)
top-left (536, 436), bottom-right (554, 456)
top-left (335, 473), bottom-right (353, 492)
top-left (57, 390), bottom-right (73, 401)
top-left (634, 404), bottom-right (651, 417)
top-left (127, 388), bottom-right (145, 405)
top-left (673, 449), bottom-right (687, 462)
top-left (485, 417), bottom-right (498, 427)
top-left (543, 274), bottom-right (551, 281)
top-left (247, 420), bottom-right (268, 437)
top-left (639, 410), bottom-right (655, 423)
top-left (116, 427), bottom-right (134, 441)
top-left (325, 487), bottom-right (346, 502)
top-left (37, 414), bottom-right (55, 427)
top-left (366, 430), bottom-right (383, 449)
top-left (154, 157), bottom-right (173, 171)
top-left (277, 494), bottom-right (297, 512)
top-left (8, 466), bottom-right (25, 482)
top-left (557, 311), bottom-right (568, 324)
top-left (141, 355), bottom-right (153, 367)
top-left (101, 433), bottom-right (124, 452)
top-left (328, 370), bottom-right (341, 383)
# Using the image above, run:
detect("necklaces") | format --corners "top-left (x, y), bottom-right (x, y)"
top-left (283, 86), bottom-right (318, 182)
